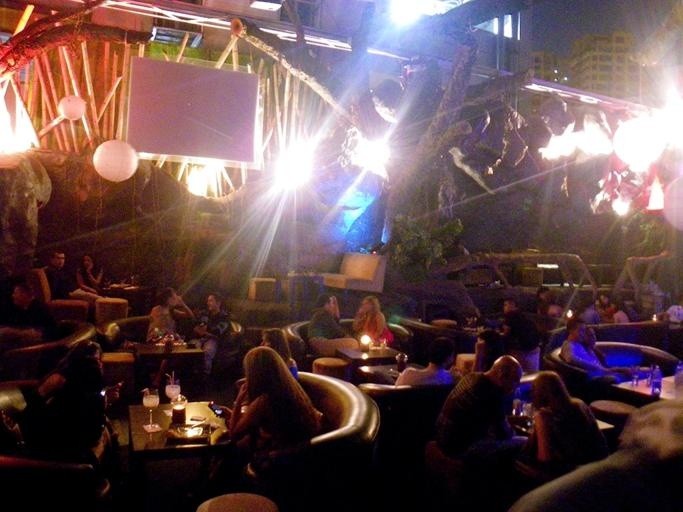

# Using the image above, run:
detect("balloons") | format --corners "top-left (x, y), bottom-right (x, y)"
top-left (93, 139), bottom-right (139, 182)
top-left (58, 96), bottom-right (85, 120)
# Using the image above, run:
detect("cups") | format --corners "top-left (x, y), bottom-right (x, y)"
top-left (512, 397), bottom-right (533, 423)
top-left (629, 361), bottom-right (683, 397)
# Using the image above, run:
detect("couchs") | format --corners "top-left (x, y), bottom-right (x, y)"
top-left (2, 252), bottom-right (682, 512)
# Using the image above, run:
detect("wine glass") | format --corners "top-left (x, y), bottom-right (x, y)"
top-left (141, 389), bottom-right (159, 430)
top-left (164, 384), bottom-right (181, 415)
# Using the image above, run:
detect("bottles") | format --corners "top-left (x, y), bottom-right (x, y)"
top-left (395, 352), bottom-right (408, 372)
top-left (171, 396), bottom-right (186, 423)
top-left (162, 334), bottom-right (175, 354)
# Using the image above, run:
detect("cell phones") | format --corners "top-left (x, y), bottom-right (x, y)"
top-left (208, 401), bottom-right (224, 417)
